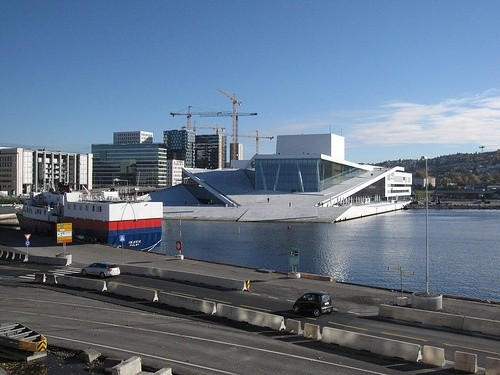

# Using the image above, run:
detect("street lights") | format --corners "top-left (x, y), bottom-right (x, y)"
top-left (421, 156), bottom-right (430, 294)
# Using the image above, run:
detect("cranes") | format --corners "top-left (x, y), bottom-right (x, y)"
top-left (216, 88), bottom-right (241, 160)
top-left (231, 130), bottom-right (273, 155)
top-left (170, 105), bottom-right (257, 132)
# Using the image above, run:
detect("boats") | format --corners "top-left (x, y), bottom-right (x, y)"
top-left (12, 181), bottom-right (163, 251)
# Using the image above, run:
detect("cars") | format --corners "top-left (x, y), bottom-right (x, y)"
top-left (81, 262), bottom-right (121, 278)
top-left (293, 293), bottom-right (332, 317)
